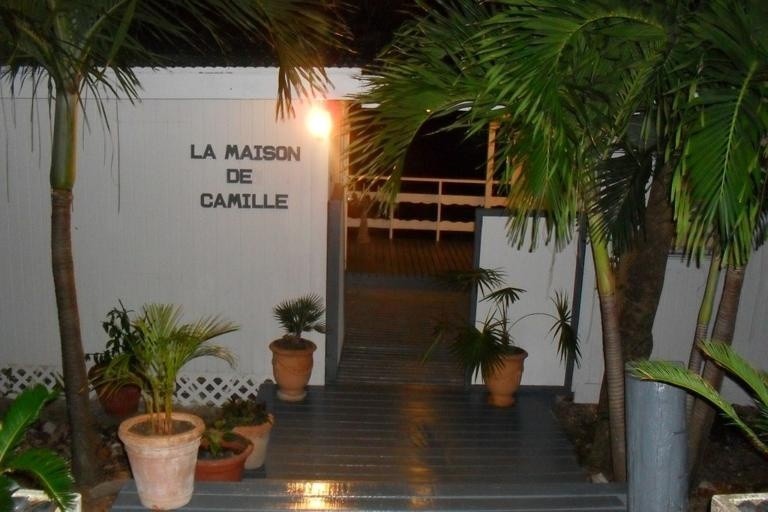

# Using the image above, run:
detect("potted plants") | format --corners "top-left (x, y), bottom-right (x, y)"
top-left (439, 266), bottom-right (585, 408)
